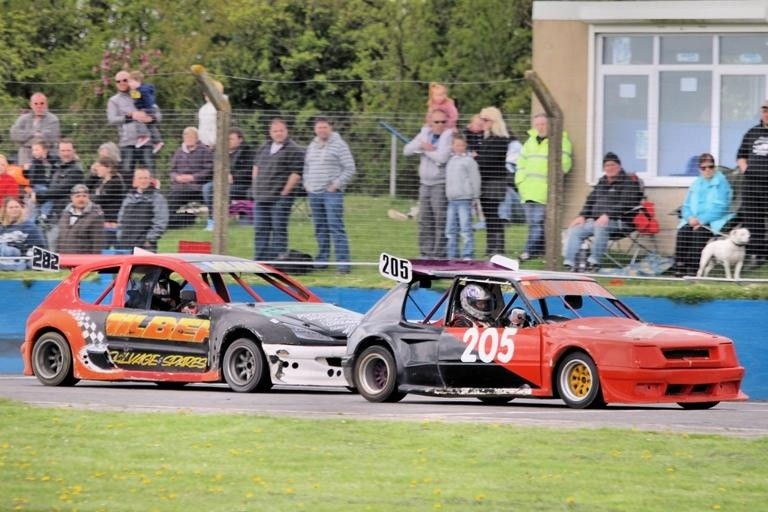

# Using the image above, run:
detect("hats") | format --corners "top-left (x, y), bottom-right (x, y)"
top-left (759, 99), bottom-right (767, 107)
top-left (603, 152), bottom-right (620, 163)
top-left (69, 184), bottom-right (89, 194)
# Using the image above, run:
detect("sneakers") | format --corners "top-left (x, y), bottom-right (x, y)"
top-left (386, 209), bottom-right (408, 223)
top-left (152, 142), bottom-right (164, 153)
top-left (135, 136), bottom-right (150, 148)
top-left (519, 252), bottom-right (530, 261)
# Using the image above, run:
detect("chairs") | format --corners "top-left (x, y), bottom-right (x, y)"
top-left (585, 176), bottom-right (659, 269)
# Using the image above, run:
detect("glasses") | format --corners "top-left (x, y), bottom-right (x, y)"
top-left (33, 102), bottom-right (44, 105)
top-left (480, 117), bottom-right (490, 121)
top-left (433, 120), bottom-right (447, 124)
top-left (700, 164), bottom-right (715, 170)
top-left (115, 79), bottom-right (127, 82)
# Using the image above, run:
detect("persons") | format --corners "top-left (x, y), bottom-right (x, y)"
top-left (197, 79), bottom-right (230, 148)
top-left (140, 274), bottom-right (175, 309)
top-left (0, 155), bottom-right (20, 197)
top-left (23, 137), bottom-right (58, 229)
top-left (479, 107), bottom-right (517, 254)
top-left (560, 152), bottom-right (645, 272)
top-left (169, 126), bottom-right (214, 202)
top-left (10, 92), bottom-right (61, 164)
top-left (451, 284), bottom-right (495, 328)
top-left (128, 70), bottom-right (165, 154)
top-left (49, 137), bottom-right (86, 207)
top-left (106, 70), bottom-right (160, 179)
top-left (302, 117), bottom-right (358, 274)
top-left (427, 80), bottom-right (457, 129)
top-left (512, 114), bottom-right (571, 261)
top-left (736, 100), bottom-right (767, 263)
top-left (445, 133), bottom-right (479, 261)
top-left (661, 154), bottom-right (734, 275)
top-left (204, 128), bottom-right (250, 232)
top-left (402, 109), bottom-right (453, 259)
top-left (56, 185), bottom-right (104, 254)
top-left (115, 166), bottom-right (169, 253)
top-left (0, 198), bottom-right (49, 254)
top-left (85, 143), bottom-right (122, 188)
top-left (97, 157), bottom-right (127, 221)
top-left (251, 116), bottom-right (304, 261)
top-left (465, 114), bottom-right (480, 150)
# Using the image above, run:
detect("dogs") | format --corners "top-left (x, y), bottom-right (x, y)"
top-left (696, 227), bottom-right (752, 280)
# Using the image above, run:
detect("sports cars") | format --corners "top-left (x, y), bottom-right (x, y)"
top-left (21, 252), bottom-right (365, 394)
top-left (341, 255), bottom-right (750, 411)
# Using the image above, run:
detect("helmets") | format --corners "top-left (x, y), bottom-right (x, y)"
top-left (459, 283), bottom-right (495, 321)
